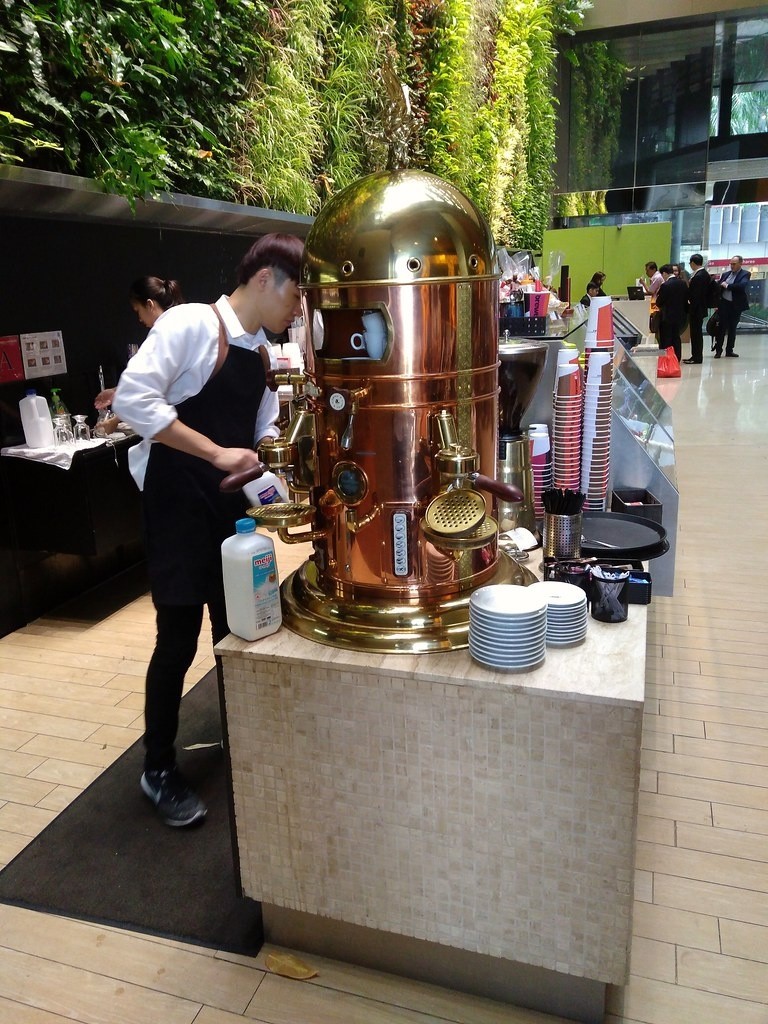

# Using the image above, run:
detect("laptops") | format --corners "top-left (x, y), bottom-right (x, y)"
top-left (627, 287), bottom-right (645, 301)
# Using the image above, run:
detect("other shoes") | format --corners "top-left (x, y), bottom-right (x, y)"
top-left (715, 348), bottom-right (723, 358)
top-left (726, 352), bottom-right (739, 357)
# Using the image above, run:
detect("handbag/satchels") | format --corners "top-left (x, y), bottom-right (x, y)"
top-left (656, 346), bottom-right (681, 378)
top-left (707, 310), bottom-right (721, 336)
top-left (650, 311), bottom-right (660, 333)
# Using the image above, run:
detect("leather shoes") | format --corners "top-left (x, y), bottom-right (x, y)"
top-left (683, 359), bottom-right (691, 361)
top-left (685, 360), bottom-right (695, 363)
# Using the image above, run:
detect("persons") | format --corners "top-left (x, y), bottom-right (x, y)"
top-left (591, 271), bottom-right (607, 296)
top-left (116, 232), bottom-right (307, 827)
top-left (682, 253), bottom-right (710, 363)
top-left (92, 274), bottom-right (187, 436)
top-left (714, 255), bottom-right (751, 358)
top-left (672, 263), bottom-right (681, 279)
top-left (656, 264), bottom-right (688, 362)
top-left (581, 281), bottom-right (599, 307)
top-left (639, 261), bottom-right (665, 302)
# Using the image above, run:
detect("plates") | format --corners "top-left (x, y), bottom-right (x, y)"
top-left (467, 579), bottom-right (587, 670)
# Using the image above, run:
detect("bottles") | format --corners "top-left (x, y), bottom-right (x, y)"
top-left (220, 518), bottom-right (282, 641)
top-left (53, 412), bottom-right (90, 448)
top-left (519, 273), bottom-right (532, 293)
top-left (19, 389), bottom-right (54, 449)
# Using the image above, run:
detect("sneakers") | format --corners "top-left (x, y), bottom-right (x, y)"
top-left (141, 770), bottom-right (209, 828)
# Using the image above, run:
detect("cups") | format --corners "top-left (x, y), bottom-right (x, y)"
top-left (527, 299), bottom-right (615, 521)
top-left (350, 313), bottom-right (389, 360)
top-left (523, 292), bottom-right (551, 317)
